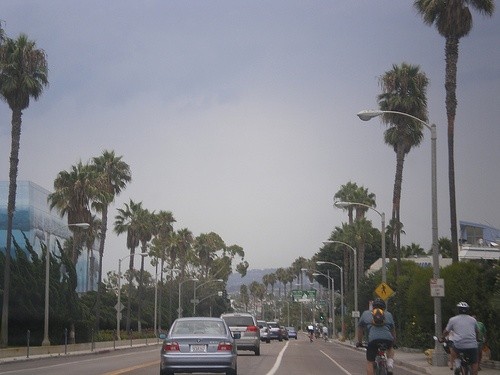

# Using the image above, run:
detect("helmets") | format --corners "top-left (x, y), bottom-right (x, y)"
top-left (372, 298), bottom-right (386, 310)
top-left (456, 301), bottom-right (470, 308)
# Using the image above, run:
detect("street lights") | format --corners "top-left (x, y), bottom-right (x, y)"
top-left (313, 273), bottom-right (337, 339)
top-left (279, 300), bottom-right (289, 327)
top-left (194, 278), bottom-right (224, 315)
top-left (41, 222), bottom-right (91, 345)
top-left (178, 278), bottom-right (199, 319)
top-left (154, 269), bottom-right (180, 338)
top-left (334, 202), bottom-right (386, 314)
top-left (322, 239), bottom-right (359, 345)
top-left (357, 111), bottom-right (447, 367)
top-left (301, 269), bottom-right (331, 337)
top-left (315, 260), bottom-right (346, 341)
top-left (116, 253), bottom-right (149, 342)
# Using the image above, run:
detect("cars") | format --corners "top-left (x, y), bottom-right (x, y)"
top-left (279, 326), bottom-right (289, 340)
top-left (220, 312), bottom-right (262, 357)
top-left (286, 327), bottom-right (298, 339)
top-left (256, 320), bottom-right (272, 344)
top-left (266, 322), bottom-right (284, 342)
top-left (160, 317), bottom-right (242, 375)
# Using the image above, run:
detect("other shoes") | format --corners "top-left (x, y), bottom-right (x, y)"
top-left (387, 362), bottom-right (394, 373)
top-left (454, 364), bottom-right (461, 375)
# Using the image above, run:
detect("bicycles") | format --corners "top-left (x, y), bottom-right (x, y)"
top-left (433, 336), bottom-right (471, 375)
top-left (308, 331), bottom-right (328, 343)
top-left (356, 342), bottom-right (399, 375)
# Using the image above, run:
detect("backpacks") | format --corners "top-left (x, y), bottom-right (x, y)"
top-left (367, 306), bottom-right (392, 335)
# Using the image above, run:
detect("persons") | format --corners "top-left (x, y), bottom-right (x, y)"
top-left (357, 297), bottom-right (397, 375)
top-left (307, 323), bottom-right (328, 343)
top-left (438, 301), bottom-right (486, 375)
